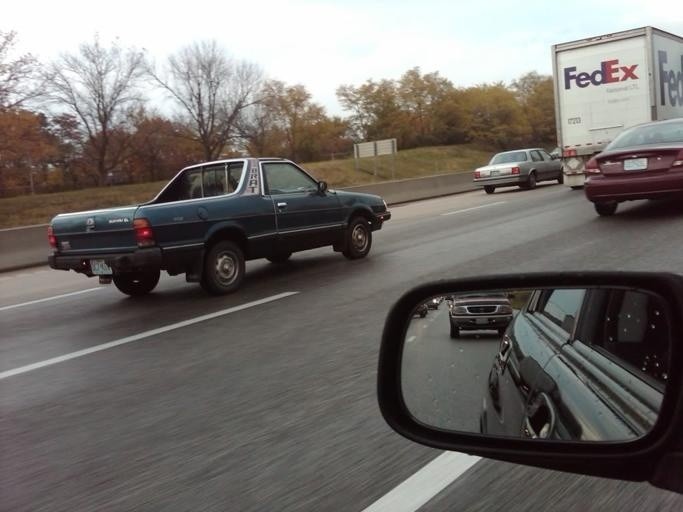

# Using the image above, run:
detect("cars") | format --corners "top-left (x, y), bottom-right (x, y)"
top-left (415, 296), bottom-right (447, 320)
top-left (581, 116), bottom-right (682, 216)
top-left (549, 147), bottom-right (558, 158)
top-left (471, 148), bottom-right (566, 193)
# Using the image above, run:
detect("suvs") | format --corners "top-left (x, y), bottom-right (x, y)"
top-left (448, 293), bottom-right (514, 339)
top-left (476, 287), bottom-right (672, 447)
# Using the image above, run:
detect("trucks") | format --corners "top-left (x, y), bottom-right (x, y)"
top-left (550, 24), bottom-right (683, 191)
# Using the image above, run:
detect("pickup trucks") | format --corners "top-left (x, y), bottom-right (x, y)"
top-left (46, 155), bottom-right (393, 301)
